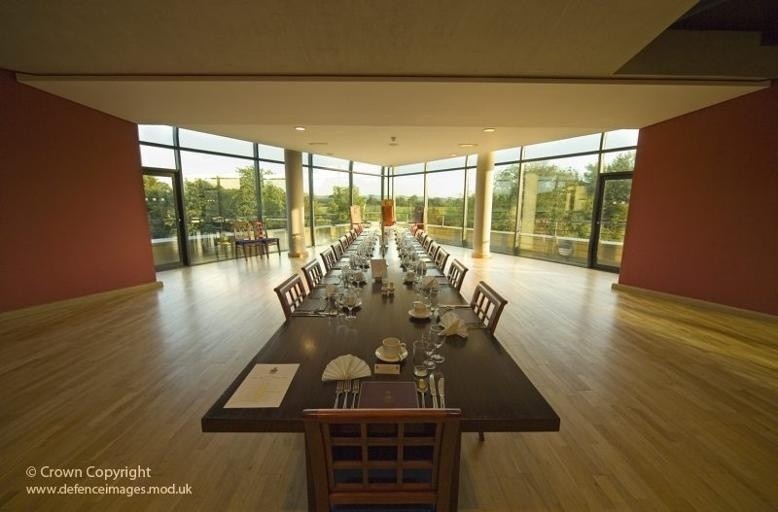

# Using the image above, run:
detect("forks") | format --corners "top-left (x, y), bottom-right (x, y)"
top-left (334, 379), bottom-right (359, 409)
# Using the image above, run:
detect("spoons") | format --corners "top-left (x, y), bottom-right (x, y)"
top-left (417, 378), bottom-right (429, 408)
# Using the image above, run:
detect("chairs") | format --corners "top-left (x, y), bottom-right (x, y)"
top-left (201, 219), bottom-right (562, 512)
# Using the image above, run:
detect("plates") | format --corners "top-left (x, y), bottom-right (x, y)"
top-left (375, 346), bottom-right (408, 363)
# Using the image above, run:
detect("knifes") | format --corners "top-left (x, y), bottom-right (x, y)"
top-left (430, 373), bottom-right (446, 408)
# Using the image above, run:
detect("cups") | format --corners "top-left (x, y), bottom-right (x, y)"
top-left (383, 338), bottom-right (405, 357)
top-left (412, 340), bottom-right (429, 377)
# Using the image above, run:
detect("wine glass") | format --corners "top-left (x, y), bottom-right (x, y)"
top-left (422, 326), bottom-right (446, 370)
top-left (326, 233), bottom-right (440, 319)
